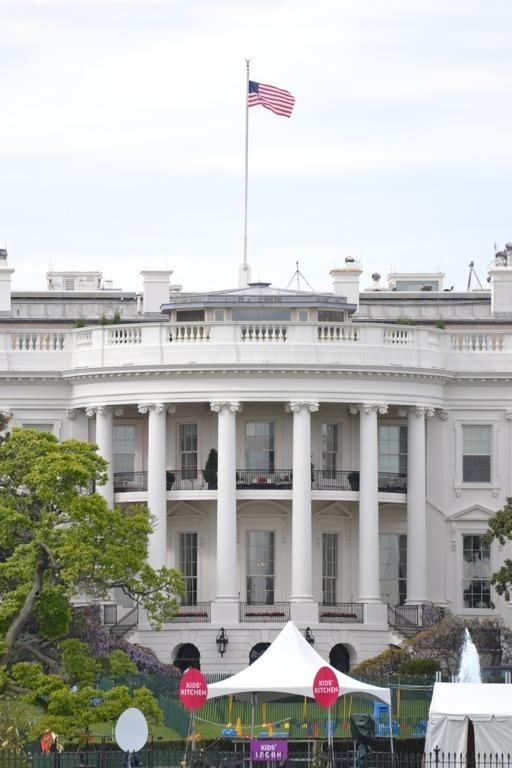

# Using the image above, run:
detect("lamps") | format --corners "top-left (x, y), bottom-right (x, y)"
top-left (304, 628), bottom-right (314, 647)
top-left (217, 631), bottom-right (227, 658)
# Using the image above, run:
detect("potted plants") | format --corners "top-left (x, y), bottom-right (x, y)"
top-left (201, 448), bottom-right (218, 489)
top-left (166, 471), bottom-right (175, 490)
top-left (347, 471), bottom-right (360, 491)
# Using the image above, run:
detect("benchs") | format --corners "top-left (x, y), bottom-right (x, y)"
top-left (319, 612), bottom-right (358, 623)
top-left (168, 612), bottom-right (208, 622)
top-left (244, 612), bottom-right (287, 622)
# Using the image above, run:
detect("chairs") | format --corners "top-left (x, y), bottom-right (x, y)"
top-left (237, 472), bottom-right (292, 490)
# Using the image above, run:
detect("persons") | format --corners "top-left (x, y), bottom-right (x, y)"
top-left (77, 728), bottom-right (90, 768)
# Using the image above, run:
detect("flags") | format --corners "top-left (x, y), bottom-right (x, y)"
top-left (247, 80), bottom-right (295, 119)
